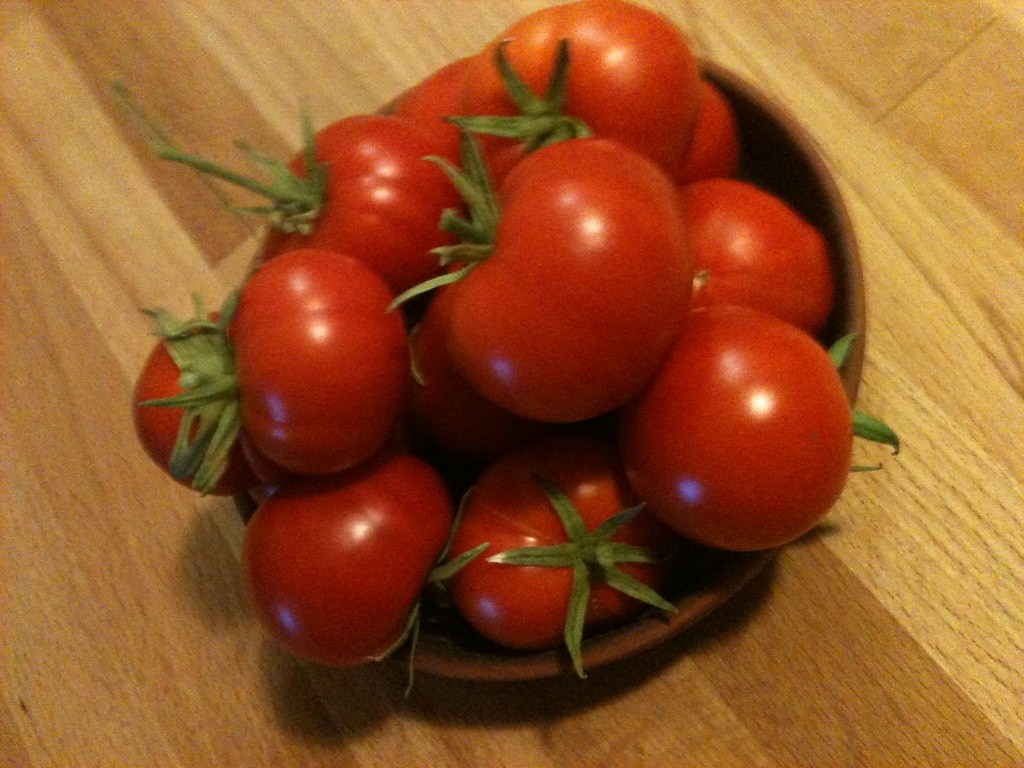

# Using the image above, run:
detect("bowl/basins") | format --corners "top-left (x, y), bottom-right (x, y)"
top-left (233, 57), bottom-right (867, 681)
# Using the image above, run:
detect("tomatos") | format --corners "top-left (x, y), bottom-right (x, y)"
top-left (128, 0), bottom-right (899, 697)
